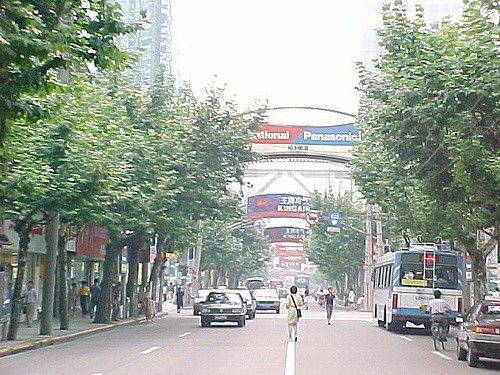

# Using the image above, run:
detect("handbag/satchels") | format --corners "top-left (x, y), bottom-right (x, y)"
top-left (297, 308), bottom-right (301, 318)
top-left (22, 296), bottom-right (27, 314)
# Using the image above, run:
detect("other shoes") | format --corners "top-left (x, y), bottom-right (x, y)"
top-left (328, 321), bottom-right (331, 325)
top-left (287, 338), bottom-right (293, 342)
top-left (295, 336), bottom-right (297, 342)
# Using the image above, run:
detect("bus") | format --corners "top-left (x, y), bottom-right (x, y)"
top-left (267, 278), bottom-right (284, 290)
top-left (244, 277), bottom-right (265, 296)
top-left (294, 273), bottom-right (309, 297)
top-left (370, 242), bottom-right (464, 332)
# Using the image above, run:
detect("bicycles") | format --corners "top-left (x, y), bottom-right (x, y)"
top-left (429, 309), bottom-right (446, 351)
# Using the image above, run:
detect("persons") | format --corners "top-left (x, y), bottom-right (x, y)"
top-left (325, 287), bottom-right (336, 325)
top-left (22, 281), bottom-right (39, 328)
top-left (286, 285), bottom-right (303, 341)
top-left (69, 280), bottom-right (184, 323)
top-left (304, 286), bottom-right (364, 309)
top-left (429, 291), bottom-right (451, 342)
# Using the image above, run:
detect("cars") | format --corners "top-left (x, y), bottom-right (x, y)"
top-left (278, 287), bottom-right (290, 299)
top-left (191, 289), bottom-right (214, 315)
top-left (238, 289), bottom-right (257, 320)
top-left (200, 289), bottom-right (247, 327)
top-left (455, 296), bottom-right (500, 367)
top-left (252, 288), bottom-right (280, 314)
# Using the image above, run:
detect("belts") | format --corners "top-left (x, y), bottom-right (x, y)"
top-left (432, 313), bottom-right (444, 315)
